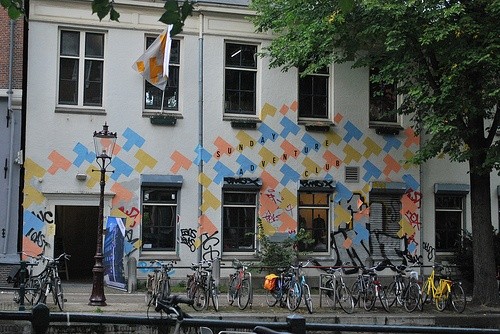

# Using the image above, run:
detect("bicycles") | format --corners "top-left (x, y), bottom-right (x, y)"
top-left (147, 291), bottom-right (293, 334)
top-left (186, 257), bottom-right (225, 312)
top-left (228, 258), bottom-right (254, 310)
top-left (145, 260), bottom-right (177, 314)
top-left (12, 252), bottom-right (71, 311)
top-left (323, 262), bottom-right (467, 314)
top-left (265, 258), bottom-right (315, 314)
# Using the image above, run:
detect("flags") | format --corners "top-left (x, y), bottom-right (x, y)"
top-left (131, 25), bottom-right (171, 90)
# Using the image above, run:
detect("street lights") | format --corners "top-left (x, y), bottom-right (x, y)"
top-left (87, 121), bottom-right (118, 306)
